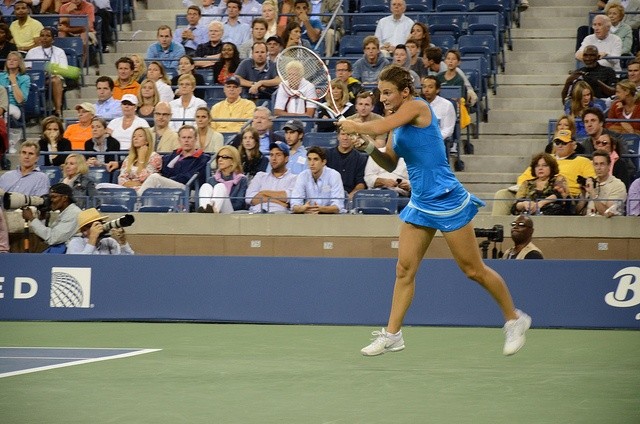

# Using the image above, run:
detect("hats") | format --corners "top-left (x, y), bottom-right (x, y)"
top-left (78, 209), bottom-right (108, 229)
top-left (553, 130), bottom-right (576, 143)
top-left (267, 35), bottom-right (282, 44)
top-left (226, 77), bottom-right (240, 85)
top-left (74, 102), bottom-right (96, 115)
top-left (50, 183), bottom-right (76, 204)
top-left (270, 141), bottom-right (289, 155)
top-left (121, 95), bottom-right (138, 105)
top-left (281, 120), bottom-right (303, 131)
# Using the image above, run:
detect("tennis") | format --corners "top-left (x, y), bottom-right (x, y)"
top-left (50, 63), bottom-right (81, 81)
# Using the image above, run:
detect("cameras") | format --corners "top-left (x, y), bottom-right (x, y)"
top-left (576, 174), bottom-right (597, 190)
top-left (99, 214), bottom-right (134, 238)
top-left (4, 191), bottom-right (51, 216)
top-left (473, 224), bottom-right (504, 243)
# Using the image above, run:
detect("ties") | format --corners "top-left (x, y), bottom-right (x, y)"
top-left (181, 28), bottom-right (194, 46)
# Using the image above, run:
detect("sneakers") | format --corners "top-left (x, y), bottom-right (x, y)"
top-left (502, 309), bottom-right (532, 357)
top-left (360, 328), bottom-right (406, 356)
top-left (1, 154), bottom-right (10, 171)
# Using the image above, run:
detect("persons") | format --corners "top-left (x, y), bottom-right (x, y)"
top-left (511, 0), bottom-right (640, 216)
top-left (337, 65), bottom-right (532, 357)
top-left (326, 36), bottom-right (411, 200)
top-left (1, 0), bottom-right (113, 122)
top-left (112, 1), bottom-right (355, 214)
top-left (377, 0), bottom-right (476, 147)
top-left (501, 215), bottom-right (545, 259)
top-left (1, 102), bottom-right (135, 253)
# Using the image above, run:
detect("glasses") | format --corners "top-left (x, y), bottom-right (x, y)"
top-left (511, 223), bottom-right (529, 228)
top-left (358, 89), bottom-right (374, 95)
top-left (336, 69), bottom-right (349, 73)
top-left (216, 155), bottom-right (232, 159)
top-left (556, 141), bottom-right (567, 145)
top-left (153, 112), bottom-right (171, 116)
top-left (595, 141), bottom-right (608, 146)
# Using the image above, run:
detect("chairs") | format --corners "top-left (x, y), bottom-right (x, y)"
top-left (303, 132), bottom-right (337, 150)
top-left (40, 166), bottom-right (61, 186)
top-left (470, 4), bottom-right (506, 70)
top-left (353, 190), bottom-right (397, 214)
top-left (479, 0), bottom-right (515, 52)
top-left (428, 24), bottom-right (457, 48)
top-left (138, 189), bottom-right (187, 213)
top-left (352, 23), bottom-right (375, 38)
top-left (338, 35), bottom-right (367, 57)
top-left (55, 37), bottom-right (85, 96)
top-left (458, 34), bottom-right (494, 123)
top-left (92, 16), bottom-right (105, 65)
top-left (355, 1), bottom-right (386, 12)
top-left (467, 23), bottom-right (498, 94)
top-left (97, 186), bottom-right (135, 213)
top-left (435, 1), bottom-right (466, 14)
top-left (460, 56), bottom-right (481, 137)
top-left (86, 168), bottom-right (110, 185)
top-left (22, 70), bottom-right (47, 125)
top-left (405, 1), bottom-right (430, 11)
